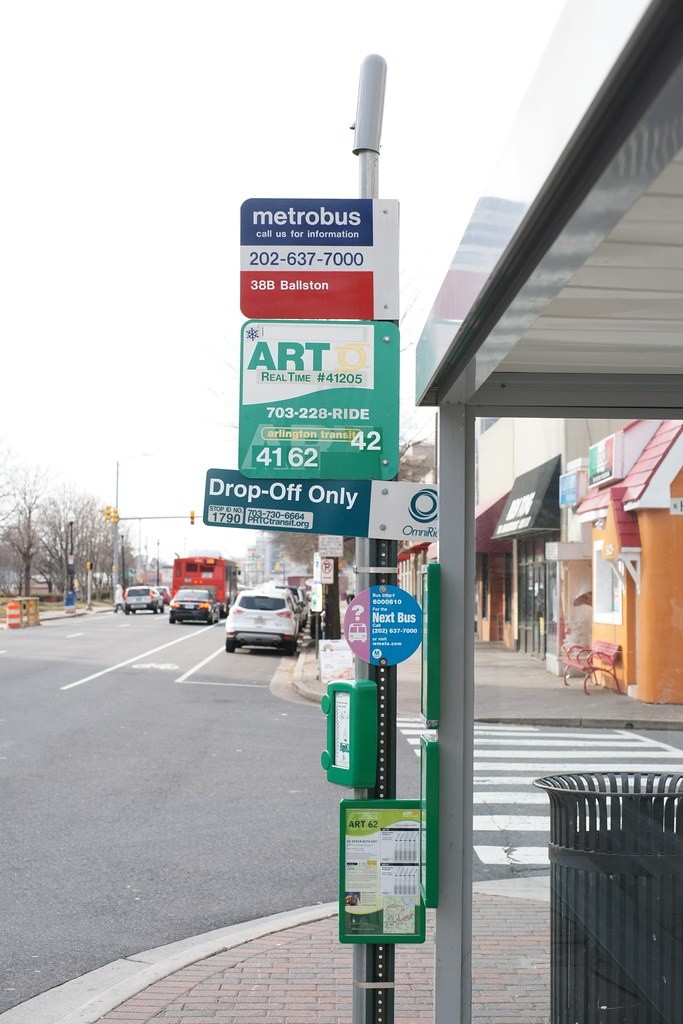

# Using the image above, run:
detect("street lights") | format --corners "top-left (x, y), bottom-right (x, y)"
top-left (118, 527), bottom-right (128, 593)
top-left (156, 537), bottom-right (161, 587)
top-left (66, 511), bottom-right (78, 613)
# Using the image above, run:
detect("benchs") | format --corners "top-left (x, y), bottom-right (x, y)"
top-left (560, 640), bottom-right (623, 696)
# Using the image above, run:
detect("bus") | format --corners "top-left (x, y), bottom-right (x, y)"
top-left (171, 558), bottom-right (242, 618)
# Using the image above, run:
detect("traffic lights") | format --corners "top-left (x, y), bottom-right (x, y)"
top-left (86, 562), bottom-right (93, 571)
top-left (105, 505), bottom-right (113, 521)
top-left (281, 552), bottom-right (285, 561)
top-left (111, 508), bottom-right (119, 525)
top-left (252, 553), bottom-right (255, 560)
top-left (191, 511), bottom-right (195, 524)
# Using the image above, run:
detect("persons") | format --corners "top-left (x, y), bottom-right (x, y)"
top-left (114, 584), bottom-right (124, 613)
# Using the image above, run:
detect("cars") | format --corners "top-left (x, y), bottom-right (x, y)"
top-left (225, 590), bottom-right (302, 657)
top-left (168, 589), bottom-right (219, 626)
top-left (155, 586), bottom-right (171, 606)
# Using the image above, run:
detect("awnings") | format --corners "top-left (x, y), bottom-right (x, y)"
top-left (428, 489), bottom-right (512, 560)
top-left (491, 455), bottom-right (561, 542)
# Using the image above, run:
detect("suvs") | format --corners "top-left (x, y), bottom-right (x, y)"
top-left (124, 586), bottom-right (164, 614)
top-left (276, 585), bottom-right (309, 631)
